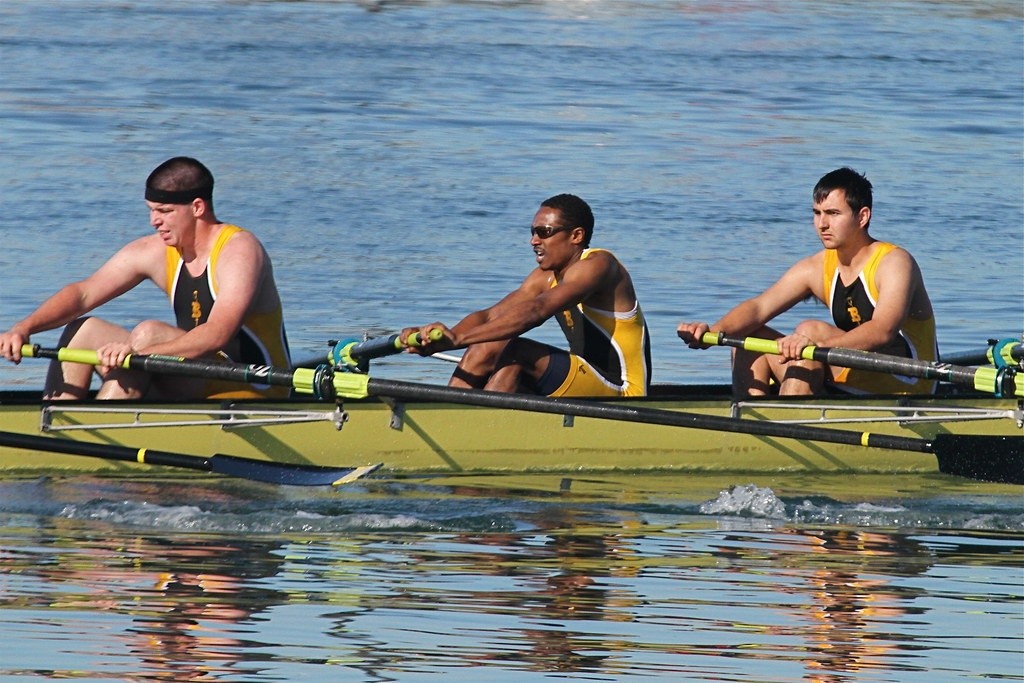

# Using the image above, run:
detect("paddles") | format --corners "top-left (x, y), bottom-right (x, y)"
top-left (290, 327), bottom-right (443, 375)
top-left (939, 337), bottom-right (1024, 371)
top-left (700, 332), bottom-right (1024, 396)
top-left (1, 431), bottom-right (384, 487)
top-left (2, 342), bottom-right (1024, 487)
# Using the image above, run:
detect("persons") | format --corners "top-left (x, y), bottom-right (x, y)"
top-left (400, 193), bottom-right (652, 397)
top-left (0, 156), bottom-right (295, 399)
top-left (677, 167), bottom-right (942, 397)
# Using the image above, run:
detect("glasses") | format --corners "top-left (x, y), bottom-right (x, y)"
top-left (530, 225), bottom-right (577, 239)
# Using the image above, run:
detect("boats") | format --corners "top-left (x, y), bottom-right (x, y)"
top-left (0, 385), bottom-right (1023, 482)
